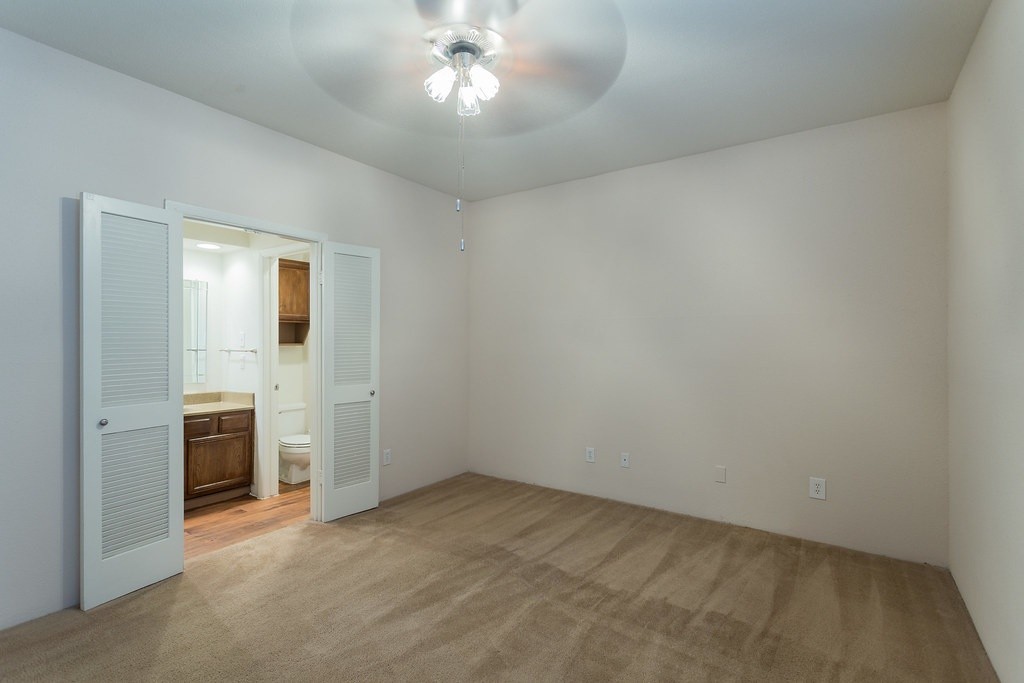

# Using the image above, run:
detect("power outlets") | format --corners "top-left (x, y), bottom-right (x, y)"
top-left (809, 477), bottom-right (826, 500)
top-left (383, 449), bottom-right (392, 466)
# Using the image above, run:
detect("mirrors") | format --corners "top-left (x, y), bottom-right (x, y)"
top-left (182, 279), bottom-right (208, 383)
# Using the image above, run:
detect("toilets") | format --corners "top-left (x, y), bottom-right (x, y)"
top-left (279, 435), bottom-right (310, 484)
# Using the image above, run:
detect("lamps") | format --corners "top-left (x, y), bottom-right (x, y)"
top-left (424, 24), bottom-right (507, 250)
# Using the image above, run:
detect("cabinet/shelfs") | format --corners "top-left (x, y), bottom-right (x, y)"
top-left (184, 408), bottom-right (254, 501)
top-left (279, 259), bottom-right (310, 323)
